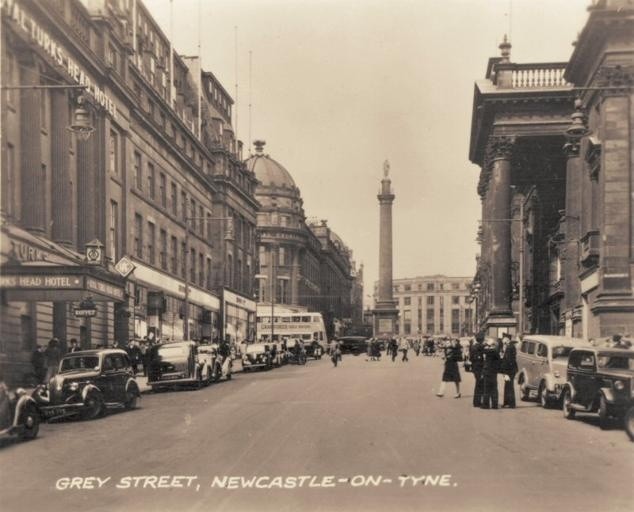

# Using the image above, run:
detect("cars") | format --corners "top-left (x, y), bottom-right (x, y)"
top-left (144, 332), bottom-right (330, 390)
top-left (561, 345), bottom-right (634, 429)
top-left (512, 335), bottom-right (596, 411)
top-left (445, 331), bottom-right (519, 374)
top-left (32, 349), bottom-right (142, 424)
top-left (1, 377), bottom-right (40, 444)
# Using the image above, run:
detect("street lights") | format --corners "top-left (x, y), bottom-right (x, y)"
top-left (475, 215), bottom-right (526, 337)
top-left (2, 81), bottom-right (102, 144)
top-left (253, 274), bottom-right (292, 344)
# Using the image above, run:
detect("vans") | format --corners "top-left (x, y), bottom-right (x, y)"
top-left (327, 337), bottom-right (371, 356)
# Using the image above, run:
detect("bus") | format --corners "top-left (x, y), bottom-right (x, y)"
top-left (255, 312), bottom-right (327, 359)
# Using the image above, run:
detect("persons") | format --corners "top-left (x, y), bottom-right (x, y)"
top-left (29, 332), bottom-right (320, 395)
top-left (328, 337), bottom-right (340, 367)
top-left (602, 333), bottom-right (630, 368)
top-left (588, 337), bottom-right (595, 347)
top-left (364, 331), bottom-right (522, 411)
